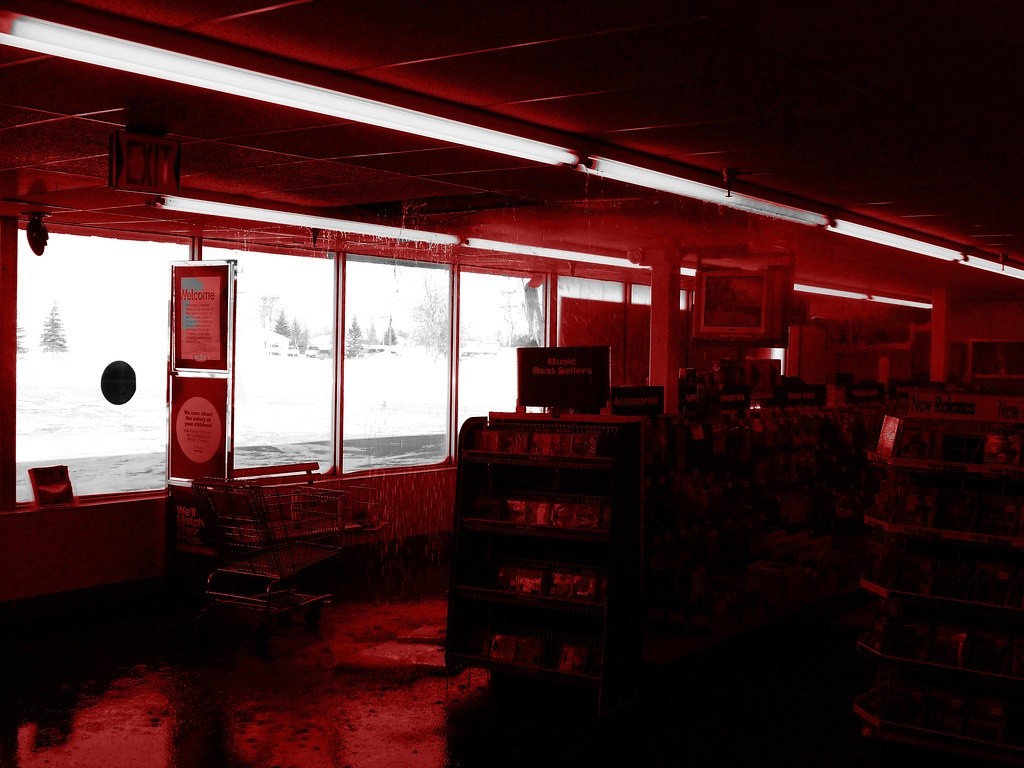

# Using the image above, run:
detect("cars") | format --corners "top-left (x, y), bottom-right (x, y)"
top-left (305, 345), bottom-right (320, 358)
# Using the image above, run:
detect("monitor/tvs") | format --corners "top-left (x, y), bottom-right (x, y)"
top-left (691, 267), bottom-right (784, 342)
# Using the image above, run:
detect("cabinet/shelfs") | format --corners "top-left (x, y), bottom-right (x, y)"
top-left (447, 395), bottom-right (1023, 767)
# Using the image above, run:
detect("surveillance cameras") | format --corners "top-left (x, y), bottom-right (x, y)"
top-left (627, 248), bottom-right (644, 264)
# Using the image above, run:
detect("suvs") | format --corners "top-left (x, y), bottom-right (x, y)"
top-left (288, 346), bottom-right (299, 356)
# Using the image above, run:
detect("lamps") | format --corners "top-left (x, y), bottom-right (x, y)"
top-left (0, 6), bottom-right (1024, 282)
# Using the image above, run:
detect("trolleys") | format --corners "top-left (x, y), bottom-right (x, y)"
top-left (190, 476), bottom-right (345, 642)
